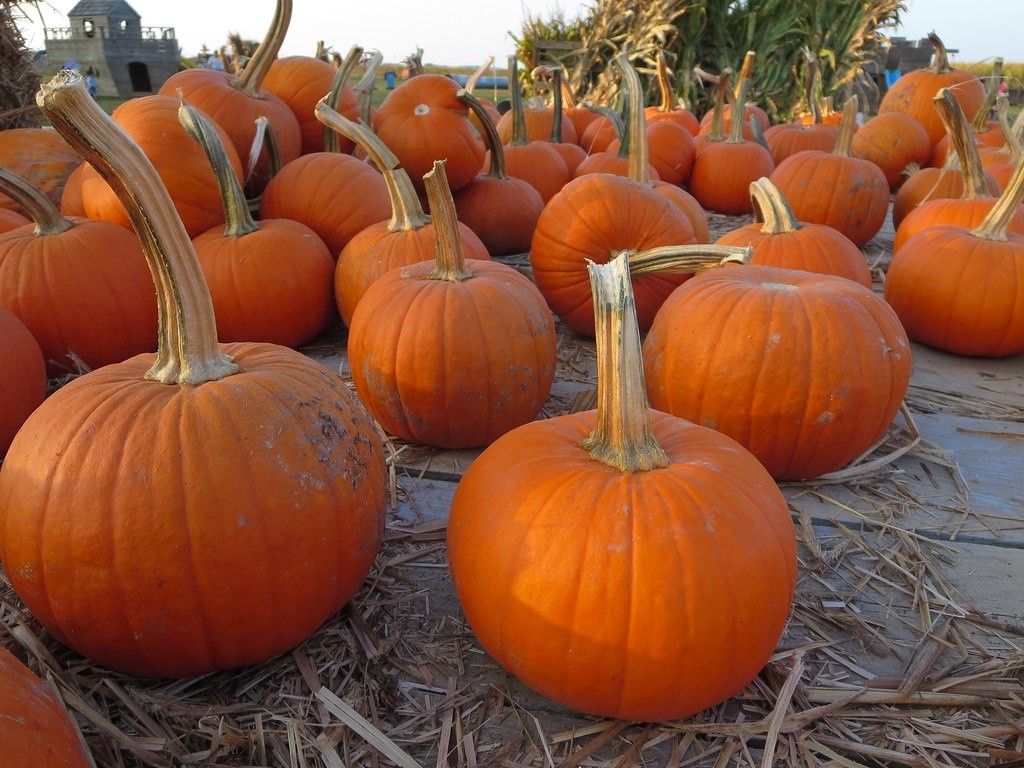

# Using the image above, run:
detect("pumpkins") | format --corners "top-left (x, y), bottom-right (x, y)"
top-left (0, 0), bottom-right (1024, 768)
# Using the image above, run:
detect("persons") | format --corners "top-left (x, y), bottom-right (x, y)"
top-left (86, 64), bottom-right (98, 98)
top-left (992, 74), bottom-right (1009, 99)
top-left (207, 49), bottom-right (224, 71)
top-left (384, 66), bottom-right (397, 88)
